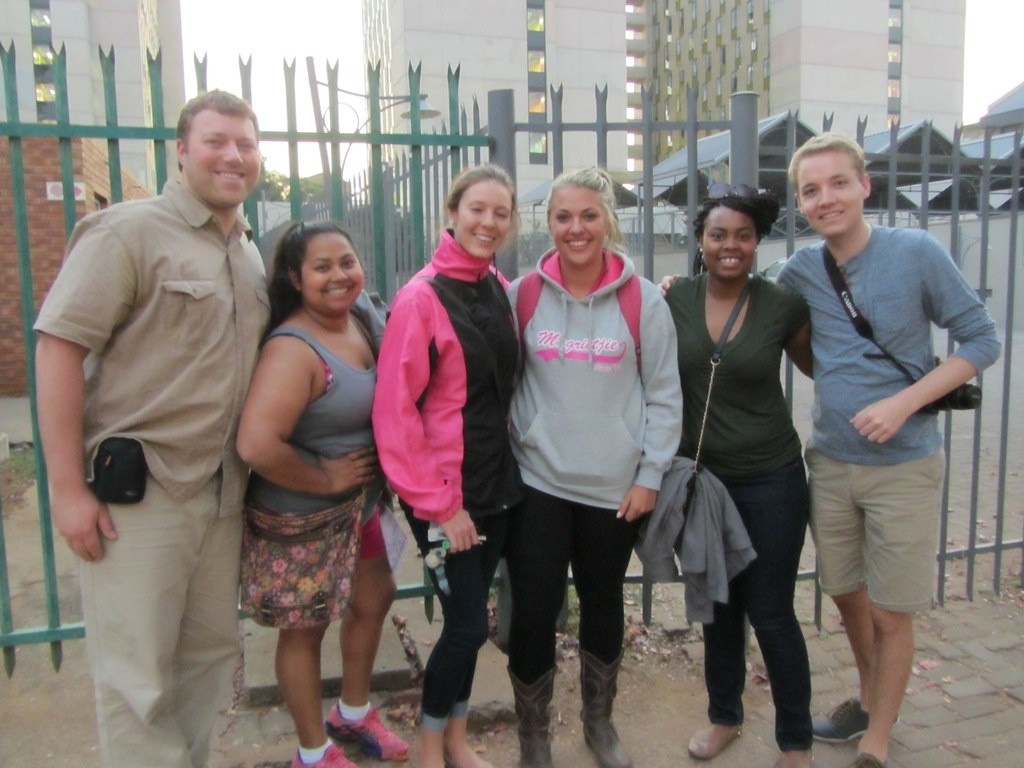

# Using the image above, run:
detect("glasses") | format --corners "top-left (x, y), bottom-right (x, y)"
top-left (706, 183), bottom-right (770, 199)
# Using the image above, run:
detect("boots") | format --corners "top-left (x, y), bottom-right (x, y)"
top-left (507, 663), bottom-right (556, 768)
top-left (578, 647), bottom-right (632, 768)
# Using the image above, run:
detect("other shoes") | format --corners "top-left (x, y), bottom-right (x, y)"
top-left (773, 748), bottom-right (812, 768)
top-left (689, 723), bottom-right (740, 758)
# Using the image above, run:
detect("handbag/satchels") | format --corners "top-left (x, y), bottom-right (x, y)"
top-left (241, 485), bottom-right (367, 631)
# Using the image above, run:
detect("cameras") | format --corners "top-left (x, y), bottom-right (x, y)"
top-left (916, 382), bottom-right (983, 417)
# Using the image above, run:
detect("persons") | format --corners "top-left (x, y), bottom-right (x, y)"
top-left (33, 91), bottom-right (1001, 768)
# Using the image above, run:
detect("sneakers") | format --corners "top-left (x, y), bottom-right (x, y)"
top-left (325, 701), bottom-right (408, 761)
top-left (290, 744), bottom-right (360, 768)
top-left (847, 752), bottom-right (889, 768)
top-left (811, 697), bottom-right (899, 742)
